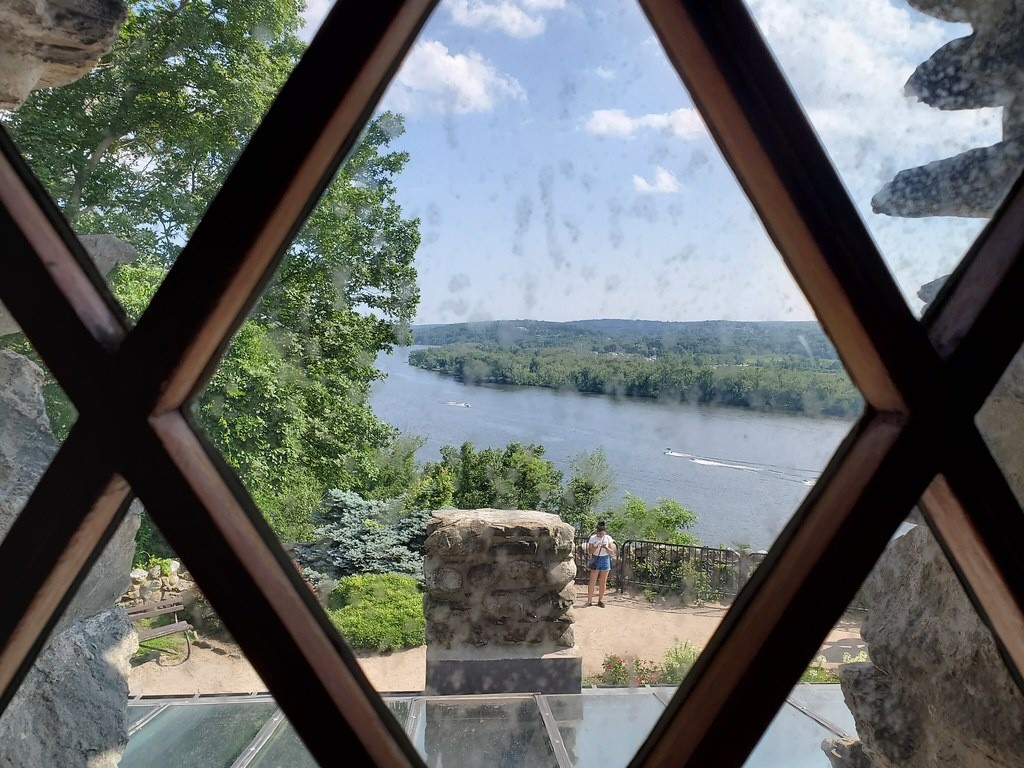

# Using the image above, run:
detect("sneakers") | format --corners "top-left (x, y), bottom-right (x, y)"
top-left (581, 602), bottom-right (592, 608)
top-left (598, 600), bottom-right (605, 608)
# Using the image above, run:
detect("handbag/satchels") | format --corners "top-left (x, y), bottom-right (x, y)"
top-left (588, 559), bottom-right (596, 570)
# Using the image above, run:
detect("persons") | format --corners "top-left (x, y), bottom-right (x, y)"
top-left (581, 521), bottom-right (614, 608)
